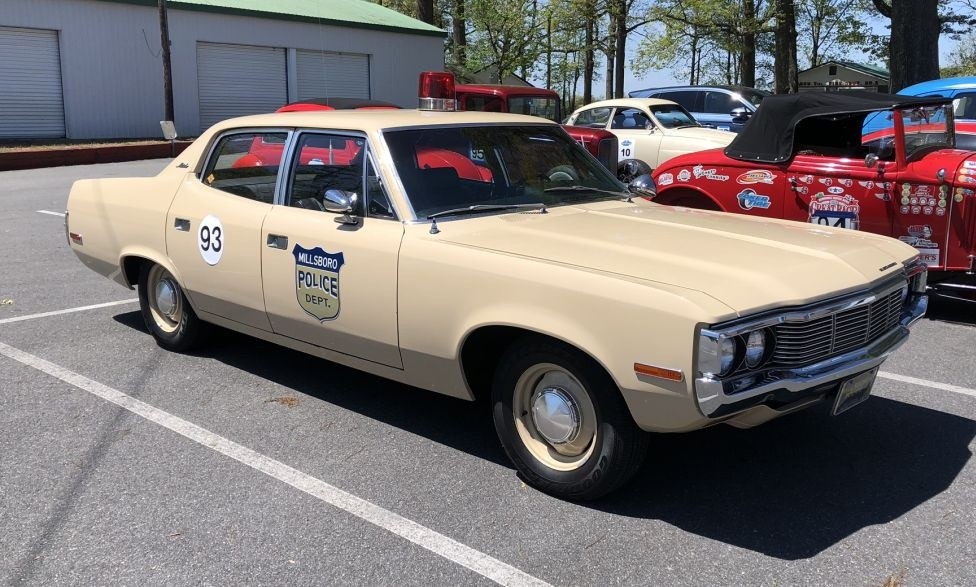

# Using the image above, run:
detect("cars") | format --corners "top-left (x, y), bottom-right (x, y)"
top-left (453, 83), bottom-right (651, 184)
top-left (561, 97), bottom-right (739, 171)
top-left (861, 75), bottom-right (976, 151)
top-left (231, 96), bottom-right (496, 184)
top-left (64, 70), bottom-right (928, 506)
top-left (644, 88), bottom-right (976, 290)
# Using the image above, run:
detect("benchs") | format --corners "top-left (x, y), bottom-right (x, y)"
top-left (218, 170), bottom-right (362, 206)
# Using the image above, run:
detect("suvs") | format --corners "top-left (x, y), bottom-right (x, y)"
top-left (622, 84), bottom-right (772, 133)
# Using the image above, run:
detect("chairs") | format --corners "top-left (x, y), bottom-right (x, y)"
top-left (413, 166), bottom-right (465, 207)
top-left (633, 112), bottom-right (649, 129)
top-left (612, 113), bottom-right (625, 129)
top-left (313, 172), bottom-right (364, 212)
